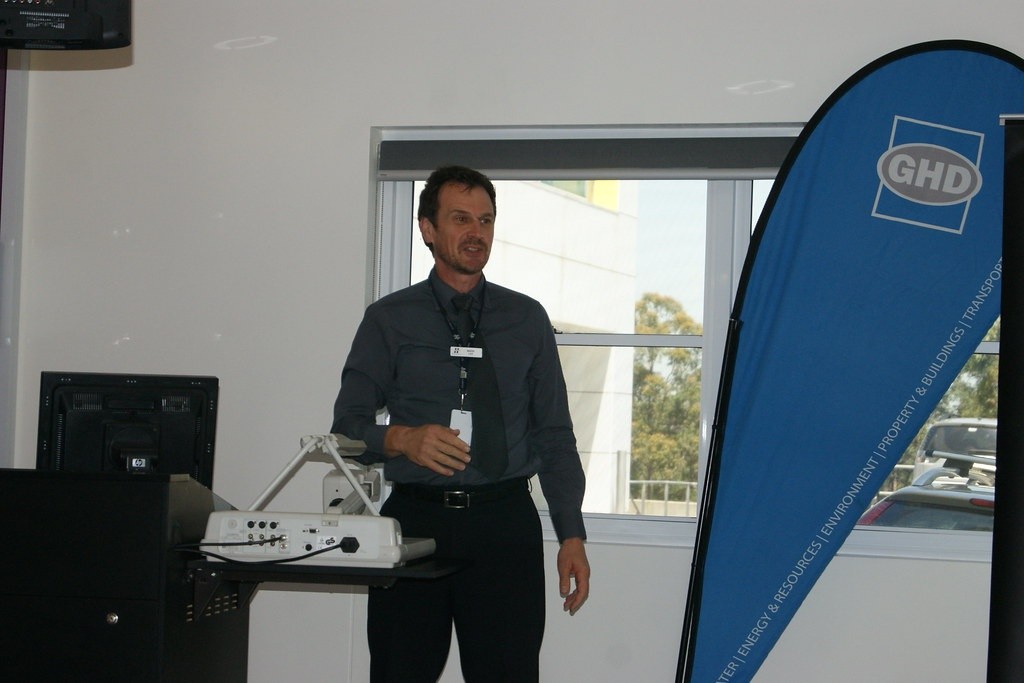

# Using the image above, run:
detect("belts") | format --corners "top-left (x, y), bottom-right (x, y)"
top-left (392, 478), bottom-right (528, 508)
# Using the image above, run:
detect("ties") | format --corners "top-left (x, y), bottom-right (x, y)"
top-left (452, 292), bottom-right (510, 482)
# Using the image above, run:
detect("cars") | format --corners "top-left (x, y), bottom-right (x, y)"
top-left (910, 418), bottom-right (996, 487)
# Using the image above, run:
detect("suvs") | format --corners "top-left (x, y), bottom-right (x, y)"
top-left (854, 466), bottom-right (994, 533)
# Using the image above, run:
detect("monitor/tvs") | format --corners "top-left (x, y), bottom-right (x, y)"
top-left (0, 0), bottom-right (132, 50)
top-left (37, 371), bottom-right (219, 489)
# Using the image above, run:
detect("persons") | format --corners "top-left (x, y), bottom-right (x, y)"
top-left (330, 166), bottom-right (591, 683)
